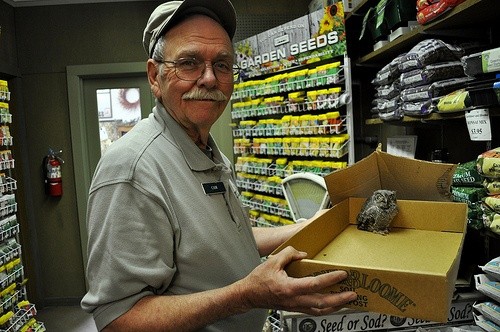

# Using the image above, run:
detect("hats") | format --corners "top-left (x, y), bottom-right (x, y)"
top-left (142, 0), bottom-right (237, 59)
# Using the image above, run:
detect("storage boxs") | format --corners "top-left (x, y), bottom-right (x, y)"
top-left (268, 198), bottom-right (468, 322)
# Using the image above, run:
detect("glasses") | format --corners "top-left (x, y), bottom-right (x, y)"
top-left (154, 57), bottom-right (242, 84)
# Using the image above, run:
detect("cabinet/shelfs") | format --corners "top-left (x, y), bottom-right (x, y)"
top-left (231, 0), bottom-right (500, 332)
top-left (0, 80), bottom-right (47, 332)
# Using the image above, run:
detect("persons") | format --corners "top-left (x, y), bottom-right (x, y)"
top-left (79, 0), bottom-right (359, 332)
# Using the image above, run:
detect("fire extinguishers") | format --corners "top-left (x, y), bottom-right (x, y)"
top-left (42, 148), bottom-right (64, 197)
